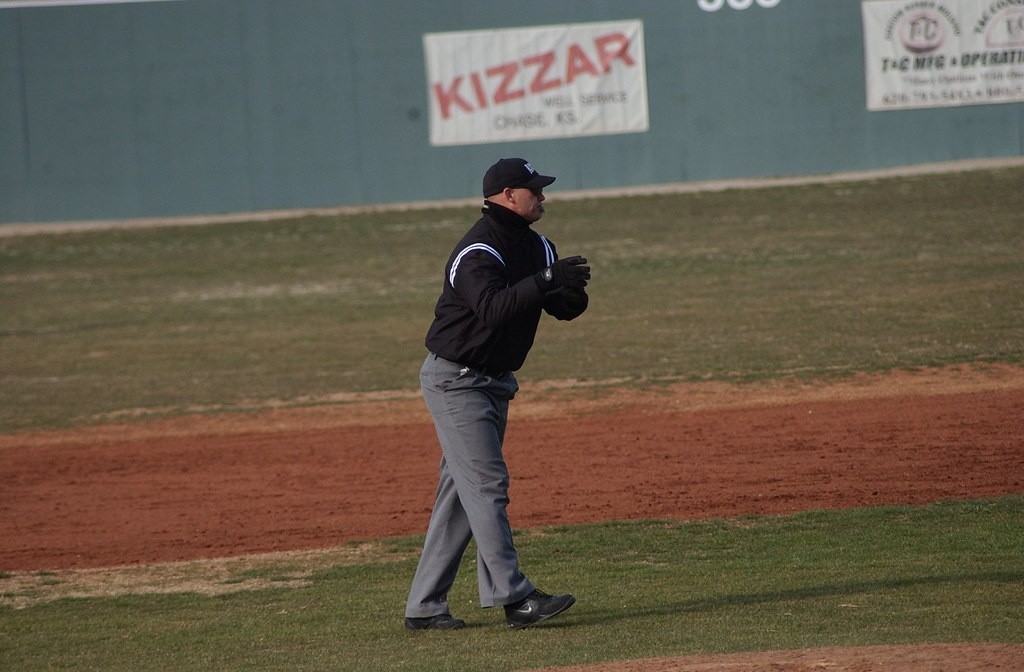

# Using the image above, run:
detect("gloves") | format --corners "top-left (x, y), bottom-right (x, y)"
top-left (534, 254), bottom-right (592, 291)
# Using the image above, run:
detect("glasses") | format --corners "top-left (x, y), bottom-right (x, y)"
top-left (528, 186), bottom-right (543, 197)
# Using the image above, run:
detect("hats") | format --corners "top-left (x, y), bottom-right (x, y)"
top-left (481, 158), bottom-right (556, 199)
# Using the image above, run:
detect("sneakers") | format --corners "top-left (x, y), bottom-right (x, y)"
top-left (502, 588), bottom-right (577, 631)
top-left (404, 614), bottom-right (466, 630)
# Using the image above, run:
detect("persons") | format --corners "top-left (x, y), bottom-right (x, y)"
top-left (404, 156), bottom-right (592, 632)
